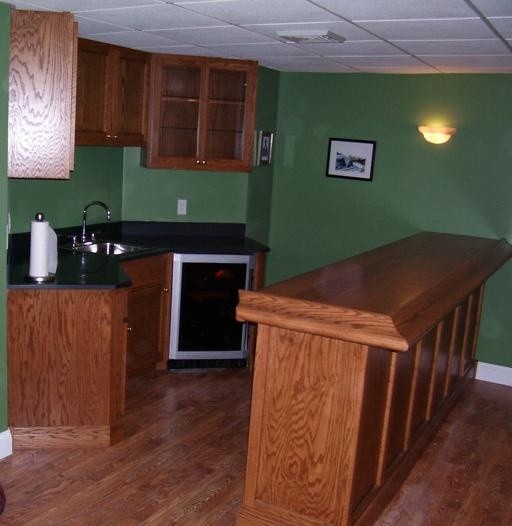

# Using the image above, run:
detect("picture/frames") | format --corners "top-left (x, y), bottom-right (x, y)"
top-left (256, 130), bottom-right (274, 166)
top-left (325, 137), bottom-right (376, 181)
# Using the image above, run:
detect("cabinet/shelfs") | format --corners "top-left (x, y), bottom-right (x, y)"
top-left (9, 252), bottom-right (172, 450)
top-left (8, 10), bottom-right (258, 181)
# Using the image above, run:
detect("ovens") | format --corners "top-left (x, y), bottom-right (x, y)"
top-left (168, 254), bottom-right (255, 370)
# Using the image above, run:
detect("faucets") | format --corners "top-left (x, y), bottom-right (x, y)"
top-left (81, 200), bottom-right (111, 243)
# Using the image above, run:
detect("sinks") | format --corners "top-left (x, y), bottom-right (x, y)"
top-left (72, 243), bottom-right (154, 257)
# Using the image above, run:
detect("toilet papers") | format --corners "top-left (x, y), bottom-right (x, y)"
top-left (29, 221), bottom-right (59, 277)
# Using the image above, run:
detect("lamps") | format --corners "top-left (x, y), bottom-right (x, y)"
top-left (418, 126), bottom-right (457, 145)
